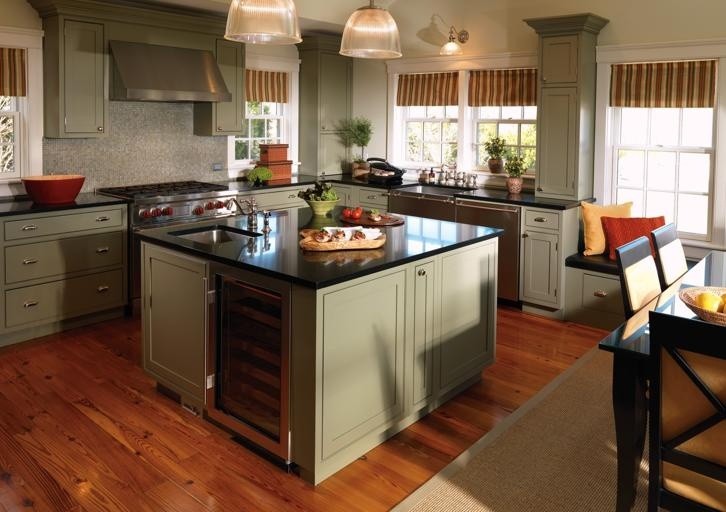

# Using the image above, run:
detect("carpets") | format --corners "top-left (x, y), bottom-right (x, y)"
top-left (385, 318), bottom-right (652, 512)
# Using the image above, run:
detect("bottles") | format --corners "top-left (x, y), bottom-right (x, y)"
top-left (429, 168), bottom-right (436, 184)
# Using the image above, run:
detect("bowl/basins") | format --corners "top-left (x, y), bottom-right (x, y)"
top-left (306, 198), bottom-right (342, 216)
top-left (21, 175), bottom-right (85, 203)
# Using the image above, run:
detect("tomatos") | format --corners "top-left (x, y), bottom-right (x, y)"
top-left (342, 206), bottom-right (362, 219)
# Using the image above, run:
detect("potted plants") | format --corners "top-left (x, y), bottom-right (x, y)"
top-left (344, 115), bottom-right (374, 180)
top-left (246, 167), bottom-right (272, 188)
top-left (503, 154), bottom-right (528, 195)
top-left (483, 138), bottom-right (508, 173)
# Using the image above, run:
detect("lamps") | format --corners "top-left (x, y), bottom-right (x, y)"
top-left (223, 0), bottom-right (303, 46)
top-left (415, 13), bottom-right (449, 47)
top-left (439, 25), bottom-right (469, 56)
top-left (339, 0), bottom-right (403, 60)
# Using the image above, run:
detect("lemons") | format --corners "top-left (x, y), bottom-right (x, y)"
top-left (694, 291), bottom-right (724, 314)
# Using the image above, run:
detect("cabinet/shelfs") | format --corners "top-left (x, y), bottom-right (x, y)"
top-left (299, 50), bottom-right (353, 176)
top-left (207, 263), bottom-right (295, 468)
top-left (534, 33), bottom-right (596, 202)
top-left (234, 183), bottom-right (311, 212)
top-left (0, 25), bottom-right (44, 202)
top-left (327, 183), bottom-right (388, 212)
top-left (434, 240), bottom-right (497, 401)
top-left (313, 266), bottom-right (407, 486)
top-left (519, 206), bottom-right (566, 310)
top-left (564, 265), bottom-right (625, 331)
top-left (0, 192), bottom-right (129, 349)
top-left (45, 15), bottom-right (108, 138)
top-left (138, 241), bottom-right (207, 416)
top-left (193, 37), bottom-right (245, 138)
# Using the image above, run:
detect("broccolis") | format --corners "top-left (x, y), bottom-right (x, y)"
top-left (248, 168), bottom-right (274, 184)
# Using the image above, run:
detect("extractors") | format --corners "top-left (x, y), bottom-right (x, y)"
top-left (107, 38), bottom-right (233, 104)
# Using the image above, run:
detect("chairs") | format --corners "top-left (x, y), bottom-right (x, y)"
top-left (650, 222), bottom-right (688, 290)
top-left (647, 309), bottom-right (726, 511)
top-left (615, 235), bottom-right (662, 465)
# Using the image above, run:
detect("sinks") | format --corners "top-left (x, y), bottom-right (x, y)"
top-left (167, 224), bottom-right (264, 246)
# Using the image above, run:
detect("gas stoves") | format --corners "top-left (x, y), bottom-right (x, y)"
top-left (96, 180), bottom-right (237, 225)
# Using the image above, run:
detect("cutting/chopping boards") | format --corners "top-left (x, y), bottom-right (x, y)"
top-left (339, 209), bottom-right (405, 226)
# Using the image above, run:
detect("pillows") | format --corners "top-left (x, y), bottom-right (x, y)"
top-left (600, 216), bottom-right (667, 259)
top-left (579, 200), bottom-right (634, 257)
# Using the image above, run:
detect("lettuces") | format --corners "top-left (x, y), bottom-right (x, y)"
top-left (322, 189), bottom-right (338, 200)
top-left (366, 208), bottom-right (379, 214)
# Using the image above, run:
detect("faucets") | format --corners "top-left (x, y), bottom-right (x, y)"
top-left (230, 238), bottom-right (258, 260)
top-left (225, 197), bottom-right (258, 228)
